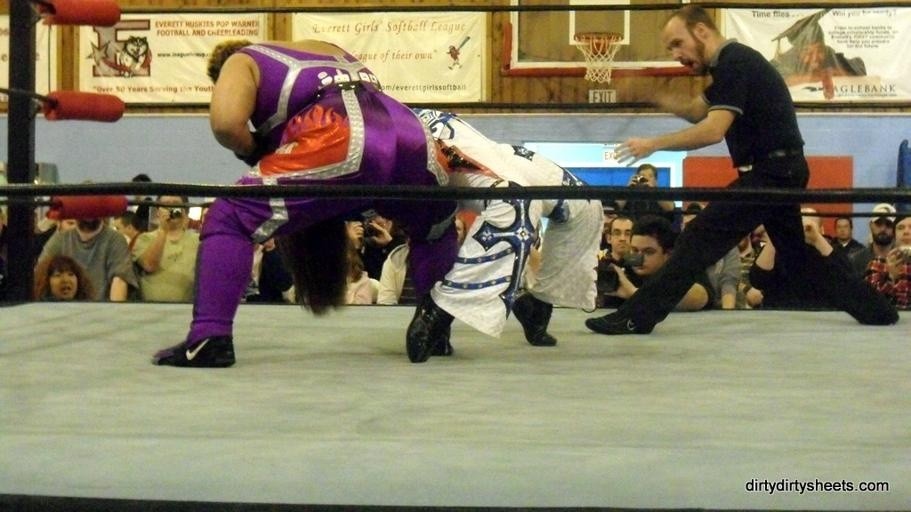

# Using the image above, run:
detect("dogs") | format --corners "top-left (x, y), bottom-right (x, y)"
top-left (119, 36), bottom-right (148, 76)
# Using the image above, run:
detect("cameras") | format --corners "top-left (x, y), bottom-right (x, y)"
top-left (632, 176), bottom-right (648, 185)
top-left (170, 208), bottom-right (183, 220)
top-left (595, 255), bottom-right (645, 293)
top-left (361, 223), bottom-right (378, 237)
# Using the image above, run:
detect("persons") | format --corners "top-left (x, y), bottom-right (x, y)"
top-left (151, 39), bottom-right (458, 368)
top-left (768, 9), bottom-right (867, 100)
top-left (292, 108), bottom-right (605, 363)
top-left (0, 164), bottom-right (911, 313)
top-left (585, 4), bottom-right (900, 334)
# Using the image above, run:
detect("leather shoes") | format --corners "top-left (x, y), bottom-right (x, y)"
top-left (510, 292), bottom-right (558, 346)
top-left (406, 292), bottom-right (455, 362)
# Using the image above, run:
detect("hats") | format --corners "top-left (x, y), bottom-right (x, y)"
top-left (869, 203), bottom-right (900, 226)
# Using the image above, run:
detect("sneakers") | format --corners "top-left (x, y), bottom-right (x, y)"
top-left (585, 308), bottom-right (656, 336)
top-left (149, 334), bottom-right (236, 368)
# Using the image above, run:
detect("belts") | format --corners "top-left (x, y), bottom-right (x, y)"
top-left (306, 82), bottom-right (384, 103)
top-left (756, 146), bottom-right (804, 159)
